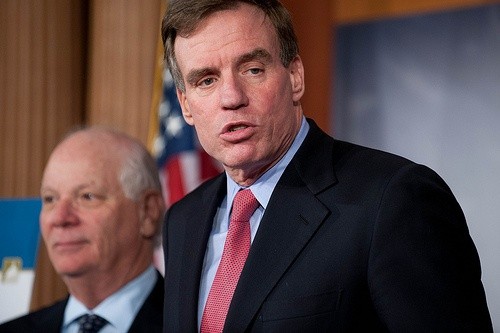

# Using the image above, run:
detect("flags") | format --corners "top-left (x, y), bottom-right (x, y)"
top-left (148, 27), bottom-right (203, 213)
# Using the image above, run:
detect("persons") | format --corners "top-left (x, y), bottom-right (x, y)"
top-left (0, 128), bottom-right (168, 333)
top-left (161, 0), bottom-right (496, 333)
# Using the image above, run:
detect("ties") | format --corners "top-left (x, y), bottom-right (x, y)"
top-left (200, 189), bottom-right (260, 333)
top-left (75, 314), bottom-right (110, 333)
